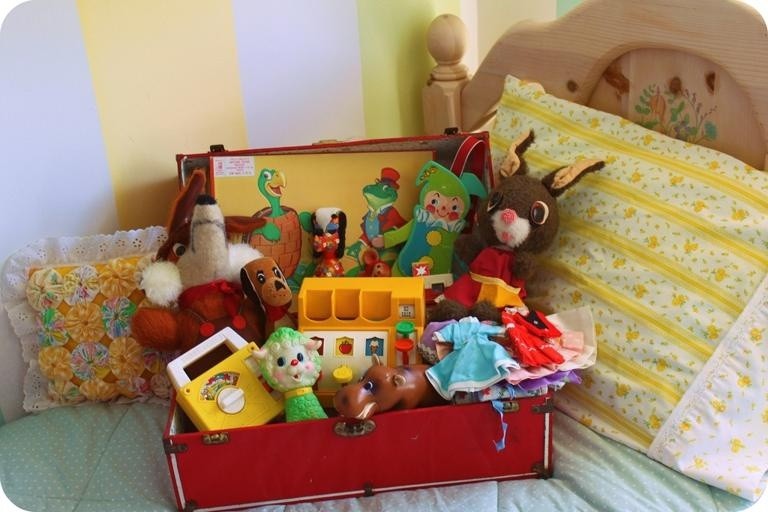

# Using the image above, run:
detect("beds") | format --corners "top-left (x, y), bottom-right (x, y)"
top-left (3, 0), bottom-right (768, 512)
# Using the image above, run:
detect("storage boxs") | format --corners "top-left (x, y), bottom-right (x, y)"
top-left (163, 130), bottom-right (555, 511)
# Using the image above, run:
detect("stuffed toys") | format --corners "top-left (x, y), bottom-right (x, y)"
top-left (427, 130), bottom-right (606, 327)
top-left (130, 169), bottom-right (293, 374)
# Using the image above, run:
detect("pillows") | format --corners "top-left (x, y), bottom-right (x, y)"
top-left (472, 74), bottom-right (768, 501)
top-left (3, 224), bottom-right (177, 411)
